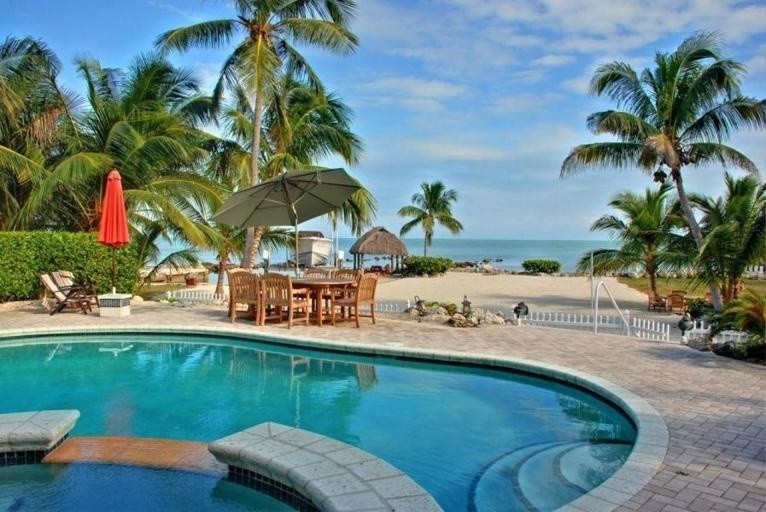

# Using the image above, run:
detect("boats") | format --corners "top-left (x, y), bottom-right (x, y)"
top-left (285, 230), bottom-right (333, 267)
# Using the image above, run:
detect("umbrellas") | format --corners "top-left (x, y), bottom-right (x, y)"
top-left (98, 167), bottom-right (131, 294)
top-left (209, 164), bottom-right (362, 279)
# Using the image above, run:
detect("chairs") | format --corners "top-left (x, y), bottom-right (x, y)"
top-left (229, 347), bottom-right (381, 393)
top-left (38, 268), bottom-right (98, 315)
top-left (225, 263), bottom-right (380, 331)
top-left (646, 288), bottom-right (712, 315)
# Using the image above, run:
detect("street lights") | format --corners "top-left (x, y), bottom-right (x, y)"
top-left (337, 250), bottom-right (346, 271)
top-left (260, 250), bottom-right (271, 273)
